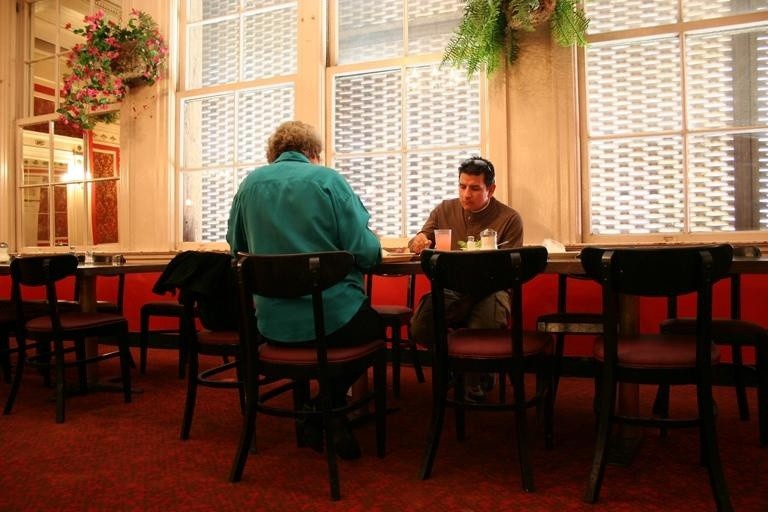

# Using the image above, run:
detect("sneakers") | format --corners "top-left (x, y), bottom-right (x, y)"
top-left (464, 373), bottom-right (496, 403)
top-left (300, 395), bottom-right (355, 458)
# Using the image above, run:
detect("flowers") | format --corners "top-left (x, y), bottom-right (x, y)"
top-left (57, 7), bottom-right (169, 134)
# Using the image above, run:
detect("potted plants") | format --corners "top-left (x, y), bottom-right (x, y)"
top-left (438, 0), bottom-right (591, 83)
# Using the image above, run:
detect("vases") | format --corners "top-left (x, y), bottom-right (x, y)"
top-left (111, 40), bottom-right (147, 83)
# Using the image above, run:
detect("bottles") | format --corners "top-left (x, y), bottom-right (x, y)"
top-left (84, 243), bottom-right (94, 264)
top-left (480, 227), bottom-right (498, 250)
top-left (68, 246), bottom-right (77, 256)
top-left (467, 236), bottom-right (475, 249)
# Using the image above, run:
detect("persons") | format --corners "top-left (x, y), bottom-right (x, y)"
top-left (227, 120), bottom-right (380, 461)
top-left (407, 156), bottom-right (523, 403)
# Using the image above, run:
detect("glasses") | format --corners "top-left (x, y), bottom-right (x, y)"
top-left (460, 158), bottom-right (493, 174)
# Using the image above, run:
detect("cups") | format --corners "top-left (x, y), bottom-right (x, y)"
top-left (433, 229), bottom-right (452, 251)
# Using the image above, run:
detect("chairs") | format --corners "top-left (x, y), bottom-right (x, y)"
top-left (651, 244), bottom-right (765, 442)
top-left (405, 278), bottom-right (512, 386)
top-left (139, 300), bottom-right (201, 384)
top-left (576, 244), bottom-right (732, 510)
top-left (420, 248), bottom-right (550, 494)
top-left (227, 251), bottom-right (391, 502)
top-left (536, 244), bottom-right (604, 429)
top-left (9, 252), bottom-right (131, 418)
top-left (156, 249), bottom-right (246, 440)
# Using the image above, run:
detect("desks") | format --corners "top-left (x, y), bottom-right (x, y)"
top-left (2, 257), bottom-right (174, 397)
top-left (366, 251), bottom-right (765, 275)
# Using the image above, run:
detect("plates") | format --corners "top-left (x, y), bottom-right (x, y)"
top-left (382, 246), bottom-right (415, 262)
top-left (549, 252), bottom-right (579, 259)
